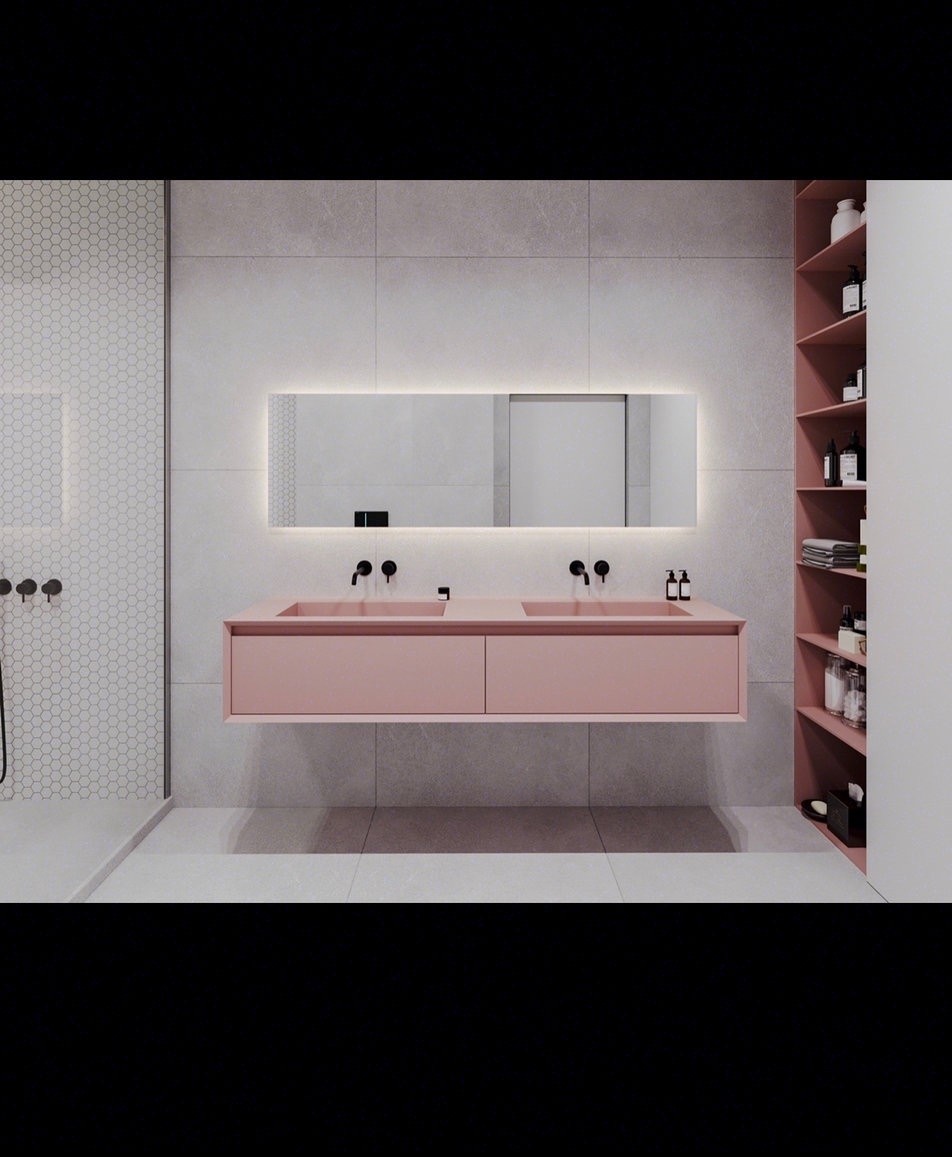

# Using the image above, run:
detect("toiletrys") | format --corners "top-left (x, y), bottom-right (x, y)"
top-left (678, 569), bottom-right (691, 601)
top-left (664, 569), bottom-right (679, 601)
top-left (823, 431), bottom-right (866, 487)
top-left (831, 197), bottom-right (867, 244)
top-left (841, 263), bottom-right (867, 318)
top-left (843, 364), bottom-right (866, 403)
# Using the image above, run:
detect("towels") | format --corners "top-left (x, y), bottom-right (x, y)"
top-left (800, 537), bottom-right (860, 569)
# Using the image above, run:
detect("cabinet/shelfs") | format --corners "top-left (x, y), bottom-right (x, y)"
top-left (218, 597), bottom-right (750, 725)
top-left (796, 180), bottom-right (871, 867)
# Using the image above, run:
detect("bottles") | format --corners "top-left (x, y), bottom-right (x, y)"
top-left (839, 605), bottom-right (867, 635)
top-left (822, 431), bottom-right (867, 487)
top-left (830, 199), bottom-right (867, 244)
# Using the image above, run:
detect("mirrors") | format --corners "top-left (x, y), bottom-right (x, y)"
top-left (257, 389), bottom-right (703, 533)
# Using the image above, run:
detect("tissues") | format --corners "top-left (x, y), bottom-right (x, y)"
top-left (828, 783), bottom-right (866, 848)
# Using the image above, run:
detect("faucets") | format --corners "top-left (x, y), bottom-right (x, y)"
top-left (352, 560), bottom-right (372, 585)
top-left (570, 560), bottom-right (590, 586)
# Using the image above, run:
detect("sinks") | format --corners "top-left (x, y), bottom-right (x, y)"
top-left (520, 599), bottom-right (694, 619)
top-left (276, 598), bottom-right (447, 619)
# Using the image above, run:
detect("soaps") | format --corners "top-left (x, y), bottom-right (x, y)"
top-left (812, 800), bottom-right (828, 815)
